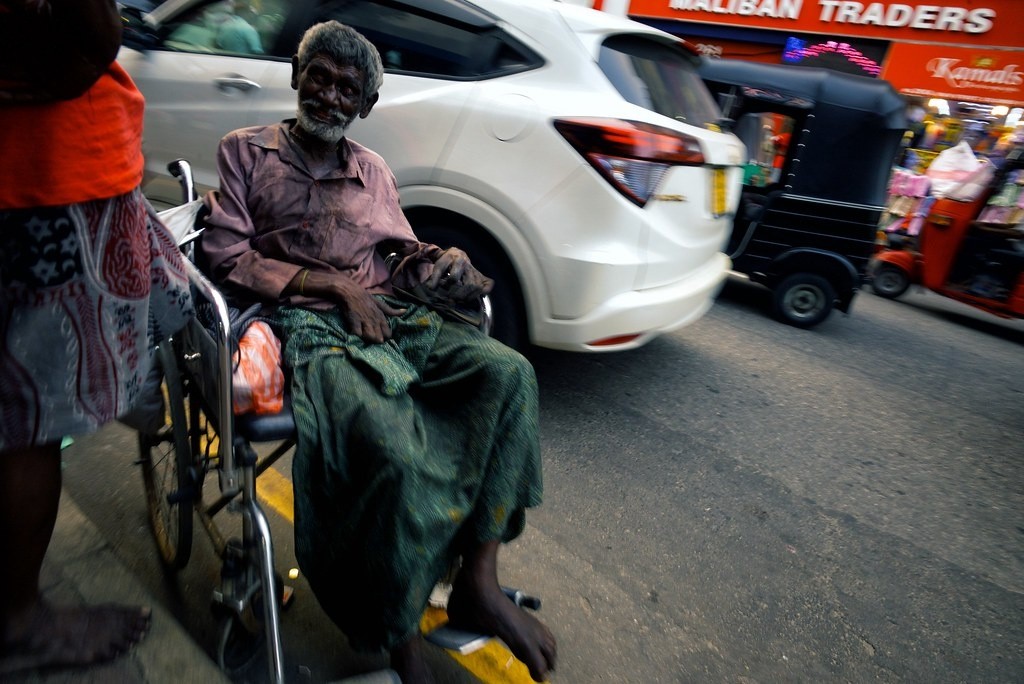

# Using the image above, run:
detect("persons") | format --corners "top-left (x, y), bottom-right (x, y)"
top-left (197, 18), bottom-right (557, 683)
top-left (0, 0), bottom-right (196, 678)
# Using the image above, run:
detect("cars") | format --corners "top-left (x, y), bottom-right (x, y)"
top-left (116, 1), bottom-right (749, 381)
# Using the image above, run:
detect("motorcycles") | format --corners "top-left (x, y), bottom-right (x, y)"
top-left (870, 143), bottom-right (1024, 321)
top-left (703, 56), bottom-right (917, 332)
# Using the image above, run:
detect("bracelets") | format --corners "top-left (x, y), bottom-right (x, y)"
top-left (300, 269), bottom-right (309, 294)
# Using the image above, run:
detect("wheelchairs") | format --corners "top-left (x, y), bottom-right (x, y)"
top-left (132, 159), bottom-right (572, 684)
